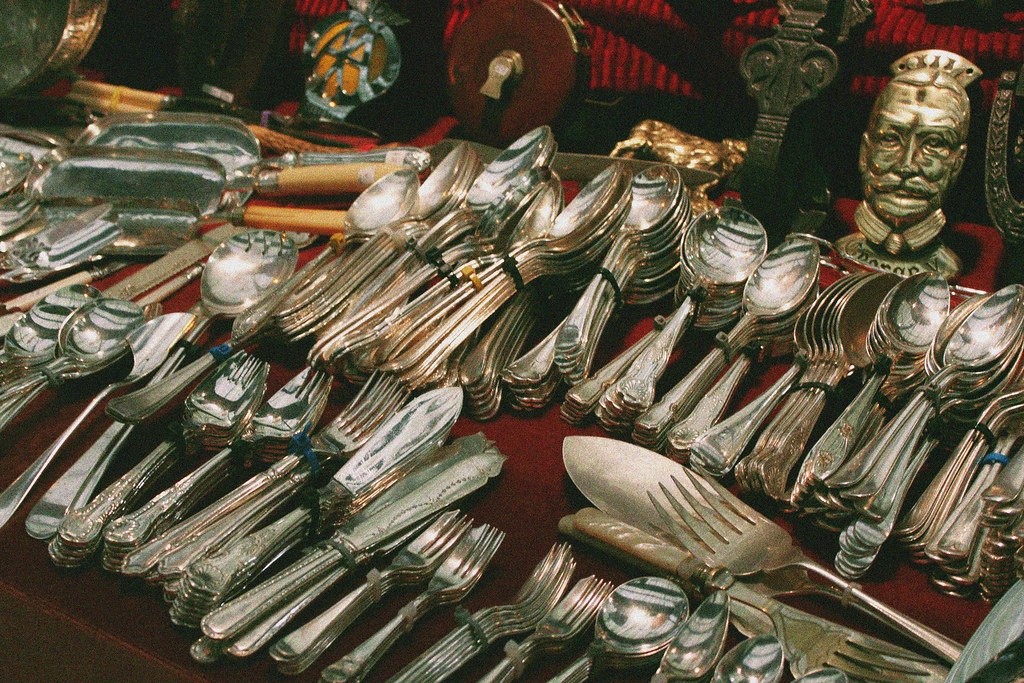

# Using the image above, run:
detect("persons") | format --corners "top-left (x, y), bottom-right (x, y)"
top-left (835, 50), bottom-right (983, 282)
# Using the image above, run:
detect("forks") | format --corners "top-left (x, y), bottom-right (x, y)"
top-left (268, 507), bottom-right (614, 683)
top-left (50, 349), bottom-right (411, 606)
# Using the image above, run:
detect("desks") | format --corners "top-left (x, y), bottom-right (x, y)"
top-left (0, 130), bottom-right (1024, 683)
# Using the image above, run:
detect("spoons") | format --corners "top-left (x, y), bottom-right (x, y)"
top-left (0, 94), bottom-right (1024, 683)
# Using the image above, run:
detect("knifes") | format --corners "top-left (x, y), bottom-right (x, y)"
top-left (646, 458), bottom-right (965, 666)
